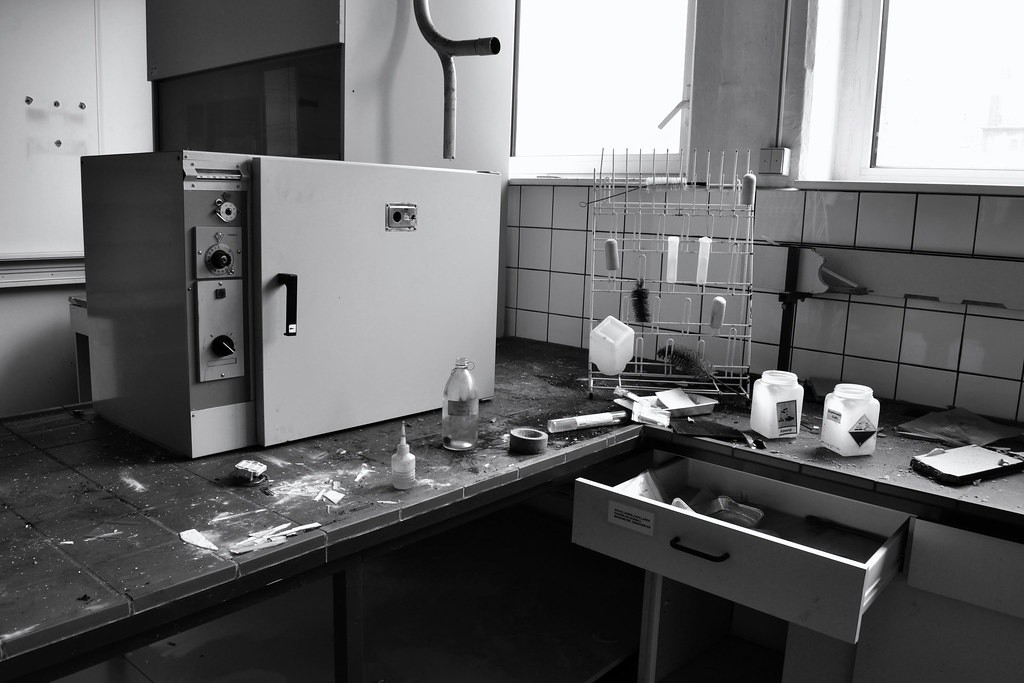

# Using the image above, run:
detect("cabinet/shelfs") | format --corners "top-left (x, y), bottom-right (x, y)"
top-left (0, 443), bottom-right (1024, 683)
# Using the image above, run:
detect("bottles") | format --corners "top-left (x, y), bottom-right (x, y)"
top-left (390, 421), bottom-right (416, 491)
top-left (749, 370), bottom-right (805, 439)
top-left (819, 383), bottom-right (880, 457)
top-left (441, 357), bottom-right (480, 451)
top-left (589, 316), bottom-right (635, 376)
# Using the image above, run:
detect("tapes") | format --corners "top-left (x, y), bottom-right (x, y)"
top-left (509, 428), bottom-right (549, 454)
